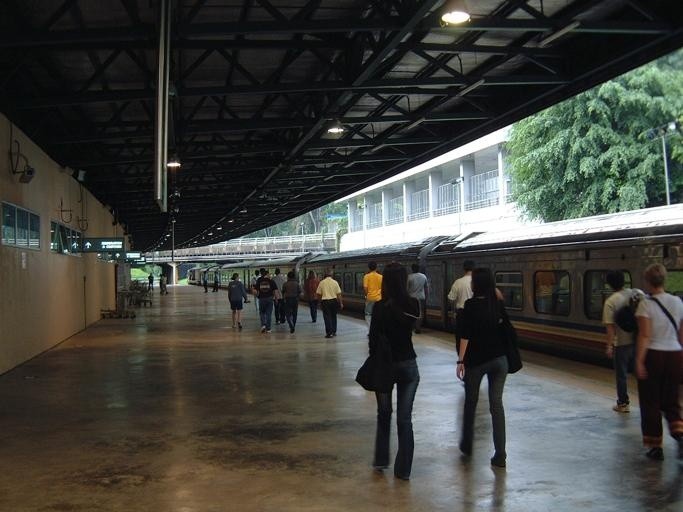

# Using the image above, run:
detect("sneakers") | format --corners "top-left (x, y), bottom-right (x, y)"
top-left (325, 332), bottom-right (336, 338)
top-left (261, 320), bottom-right (296, 333)
top-left (415, 329), bottom-right (420, 334)
top-left (613, 402), bottom-right (631, 412)
top-left (232, 321), bottom-right (243, 328)
top-left (674, 431), bottom-right (682, 446)
top-left (490, 456), bottom-right (505, 467)
top-left (645, 445), bottom-right (664, 460)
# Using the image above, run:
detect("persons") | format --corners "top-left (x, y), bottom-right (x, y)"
top-left (635, 261), bottom-right (682, 460)
top-left (202, 271), bottom-right (207, 292)
top-left (405, 262), bottom-right (428, 334)
top-left (159, 273), bottom-right (162, 293)
top-left (363, 261), bottom-right (382, 336)
top-left (147, 273), bottom-right (154, 290)
top-left (315, 269), bottom-right (344, 338)
top-left (447, 259), bottom-right (504, 357)
top-left (211, 271), bottom-right (218, 292)
top-left (455, 268), bottom-right (522, 468)
top-left (601, 270), bottom-right (647, 413)
top-left (227, 267), bottom-right (321, 334)
top-left (353, 262), bottom-right (421, 482)
top-left (160, 274), bottom-right (170, 294)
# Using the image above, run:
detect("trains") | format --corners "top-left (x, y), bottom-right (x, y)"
top-left (185, 201), bottom-right (683, 379)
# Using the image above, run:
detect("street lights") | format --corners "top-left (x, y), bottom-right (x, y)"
top-left (645, 120), bottom-right (683, 207)
top-left (299, 220), bottom-right (305, 236)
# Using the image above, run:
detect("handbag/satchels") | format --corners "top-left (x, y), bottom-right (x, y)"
top-left (355, 358), bottom-right (394, 392)
top-left (500, 324), bottom-right (523, 373)
top-left (615, 299), bottom-right (640, 332)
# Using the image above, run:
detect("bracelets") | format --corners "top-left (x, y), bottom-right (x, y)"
top-left (456, 360), bottom-right (464, 364)
